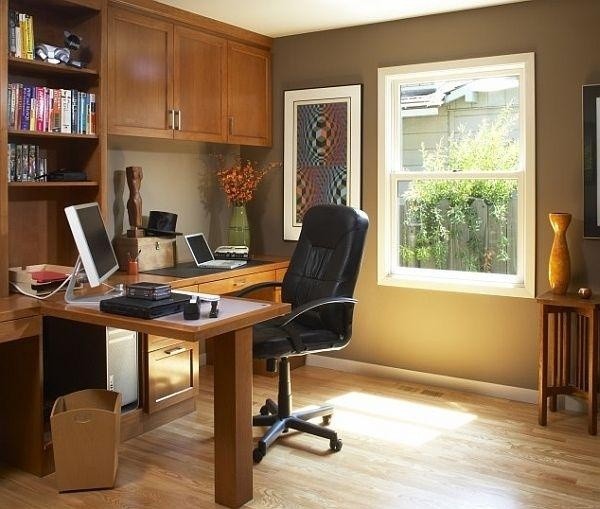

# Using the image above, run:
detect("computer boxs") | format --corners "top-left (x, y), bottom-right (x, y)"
top-left (42, 317), bottom-right (139, 417)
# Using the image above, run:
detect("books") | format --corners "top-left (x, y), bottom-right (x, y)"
top-left (6, 8), bottom-right (98, 183)
top-left (27, 271), bottom-right (69, 284)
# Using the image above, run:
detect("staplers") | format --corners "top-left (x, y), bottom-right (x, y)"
top-left (209, 301), bottom-right (218, 318)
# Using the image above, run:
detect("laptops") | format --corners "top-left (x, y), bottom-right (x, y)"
top-left (185, 233), bottom-right (247, 269)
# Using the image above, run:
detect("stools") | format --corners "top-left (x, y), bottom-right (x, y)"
top-left (535, 286), bottom-right (599, 436)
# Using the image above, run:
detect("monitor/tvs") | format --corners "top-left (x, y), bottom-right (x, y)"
top-left (64, 202), bottom-right (122, 307)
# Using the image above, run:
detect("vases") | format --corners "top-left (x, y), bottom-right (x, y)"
top-left (547, 212), bottom-right (573, 294)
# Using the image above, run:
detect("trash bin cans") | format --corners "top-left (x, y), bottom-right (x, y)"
top-left (49, 388), bottom-right (122, 493)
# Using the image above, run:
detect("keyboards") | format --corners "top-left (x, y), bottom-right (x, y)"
top-left (171, 287), bottom-right (220, 303)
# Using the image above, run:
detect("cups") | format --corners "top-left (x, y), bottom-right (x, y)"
top-left (576, 288), bottom-right (592, 299)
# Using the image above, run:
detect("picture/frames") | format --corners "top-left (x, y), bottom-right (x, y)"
top-left (581, 84), bottom-right (600, 240)
top-left (281, 83), bottom-right (364, 241)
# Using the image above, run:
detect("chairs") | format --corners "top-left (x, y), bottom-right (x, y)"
top-left (232, 203), bottom-right (370, 463)
